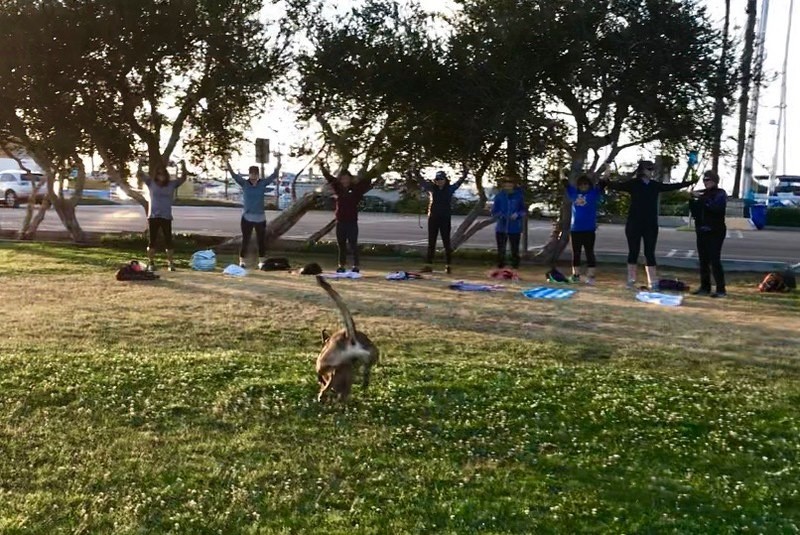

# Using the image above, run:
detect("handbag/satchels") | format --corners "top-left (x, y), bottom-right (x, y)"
top-left (189, 249), bottom-right (217, 272)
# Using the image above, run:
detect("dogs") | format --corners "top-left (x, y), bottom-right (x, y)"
top-left (310, 274), bottom-right (379, 405)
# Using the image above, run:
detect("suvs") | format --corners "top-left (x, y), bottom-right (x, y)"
top-left (0, 170), bottom-right (47, 208)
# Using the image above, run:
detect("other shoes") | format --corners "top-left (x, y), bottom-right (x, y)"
top-left (692, 289), bottom-right (713, 295)
top-left (148, 261), bottom-right (156, 271)
top-left (416, 265), bottom-right (433, 273)
top-left (168, 261), bottom-right (175, 271)
top-left (239, 257), bottom-right (246, 268)
top-left (352, 266), bottom-right (359, 273)
top-left (647, 283), bottom-right (660, 292)
top-left (585, 273), bottom-right (595, 285)
top-left (336, 267), bottom-right (346, 273)
top-left (711, 290), bottom-right (727, 297)
top-left (626, 278), bottom-right (635, 289)
top-left (445, 264), bottom-right (451, 274)
top-left (571, 273), bottom-right (581, 284)
top-left (258, 262), bottom-right (264, 271)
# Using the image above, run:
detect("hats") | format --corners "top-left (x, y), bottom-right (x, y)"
top-left (300, 262), bottom-right (323, 275)
top-left (436, 171), bottom-right (446, 178)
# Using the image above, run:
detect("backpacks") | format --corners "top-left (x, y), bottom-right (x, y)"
top-left (658, 277), bottom-right (690, 292)
top-left (758, 273), bottom-right (791, 292)
top-left (258, 256), bottom-right (291, 271)
top-left (546, 269), bottom-right (569, 285)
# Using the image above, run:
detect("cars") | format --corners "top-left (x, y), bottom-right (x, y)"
top-left (527, 200), bottom-right (560, 219)
top-left (263, 186), bottom-right (277, 199)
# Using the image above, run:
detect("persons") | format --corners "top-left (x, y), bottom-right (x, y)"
top-left (490, 173), bottom-right (527, 283)
top-left (226, 160), bottom-right (283, 269)
top-left (689, 171), bottom-right (727, 298)
top-left (608, 160), bottom-right (698, 292)
top-left (318, 162), bottom-right (370, 275)
top-left (560, 170), bottom-right (610, 284)
top-left (413, 166), bottom-right (468, 274)
top-left (138, 160), bottom-right (187, 273)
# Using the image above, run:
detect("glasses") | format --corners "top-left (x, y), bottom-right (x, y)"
top-left (703, 179), bottom-right (710, 182)
top-left (647, 167), bottom-right (653, 171)
top-left (504, 180), bottom-right (513, 183)
top-left (578, 182), bottom-right (587, 186)
top-left (435, 178), bottom-right (444, 181)
